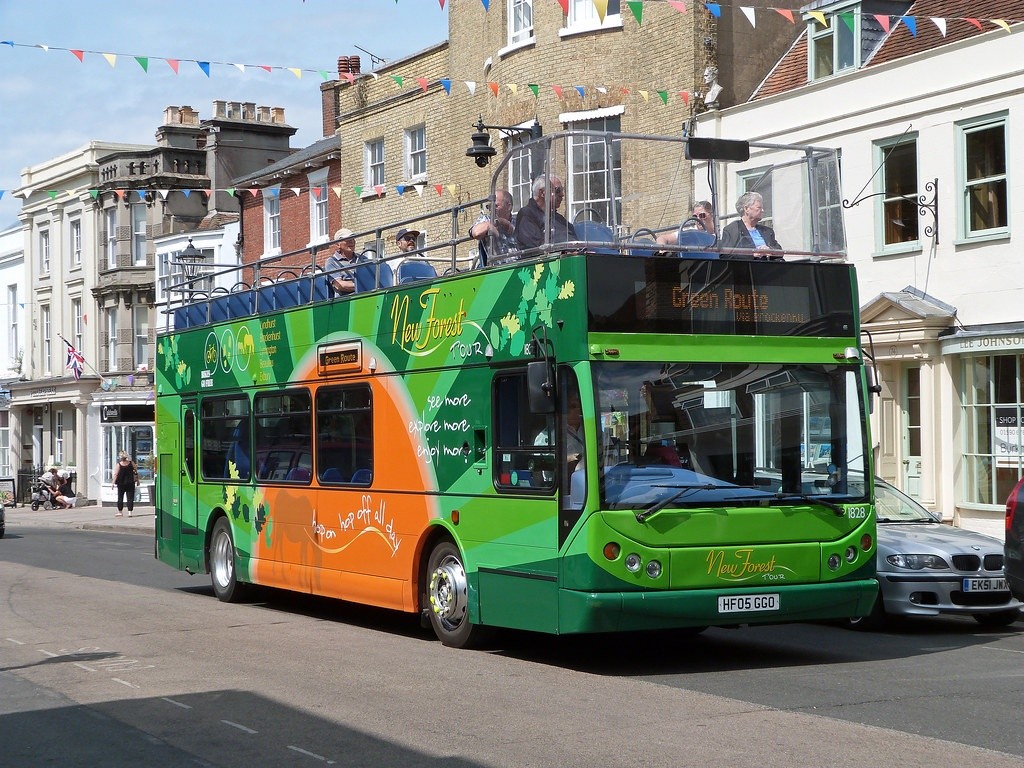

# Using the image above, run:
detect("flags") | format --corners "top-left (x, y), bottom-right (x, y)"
top-left (65, 346), bottom-right (85, 381)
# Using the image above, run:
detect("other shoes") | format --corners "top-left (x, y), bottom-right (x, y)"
top-left (128, 511), bottom-right (133, 516)
top-left (115, 511), bottom-right (123, 516)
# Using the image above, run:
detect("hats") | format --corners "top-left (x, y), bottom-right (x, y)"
top-left (335, 229), bottom-right (355, 241)
top-left (395, 228), bottom-right (420, 242)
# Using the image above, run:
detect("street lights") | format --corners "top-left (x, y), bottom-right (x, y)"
top-left (176, 237), bottom-right (206, 302)
top-left (466, 111), bottom-right (544, 199)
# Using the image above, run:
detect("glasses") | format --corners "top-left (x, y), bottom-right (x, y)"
top-left (555, 187), bottom-right (564, 193)
top-left (401, 236), bottom-right (417, 242)
top-left (691, 212), bottom-right (711, 219)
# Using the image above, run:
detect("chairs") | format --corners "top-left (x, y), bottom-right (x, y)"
top-left (223, 416), bottom-right (372, 484)
top-left (173, 210), bottom-right (720, 329)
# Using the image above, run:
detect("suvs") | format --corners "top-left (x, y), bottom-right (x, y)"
top-left (254, 434), bottom-right (372, 481)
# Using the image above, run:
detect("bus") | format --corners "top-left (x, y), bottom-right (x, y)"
top-left (149, 129), bottom-right (886, 651)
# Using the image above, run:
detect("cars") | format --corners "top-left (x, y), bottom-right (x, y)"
top-left (750, 465), bottom-right (1024, 633)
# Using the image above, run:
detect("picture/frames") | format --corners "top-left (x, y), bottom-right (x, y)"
top-left (136, 428), bottom-right (152, 479)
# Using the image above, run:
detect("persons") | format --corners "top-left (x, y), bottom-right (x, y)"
top-left (111, 450), bottom-right (140, 517)
top-left (387, 228), bottom-right (429, 286)
top-left (722, 192), bottom-right (782, 259)
top-left (325, 229), bottom-right (367, 298)
top-left (42, 469), bottom-right (76, 510)
top-left (533, 388), bottom-right (619, 471)
top-left (515, 173), bottom-right (579, 254)
top-left (656, 201), bottom-right (715, 253)
top-left (469, 189), bottom-right (521, 266)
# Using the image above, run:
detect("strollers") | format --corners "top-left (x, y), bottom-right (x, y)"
top-left (27, 477), bottom-right (67, 512)
